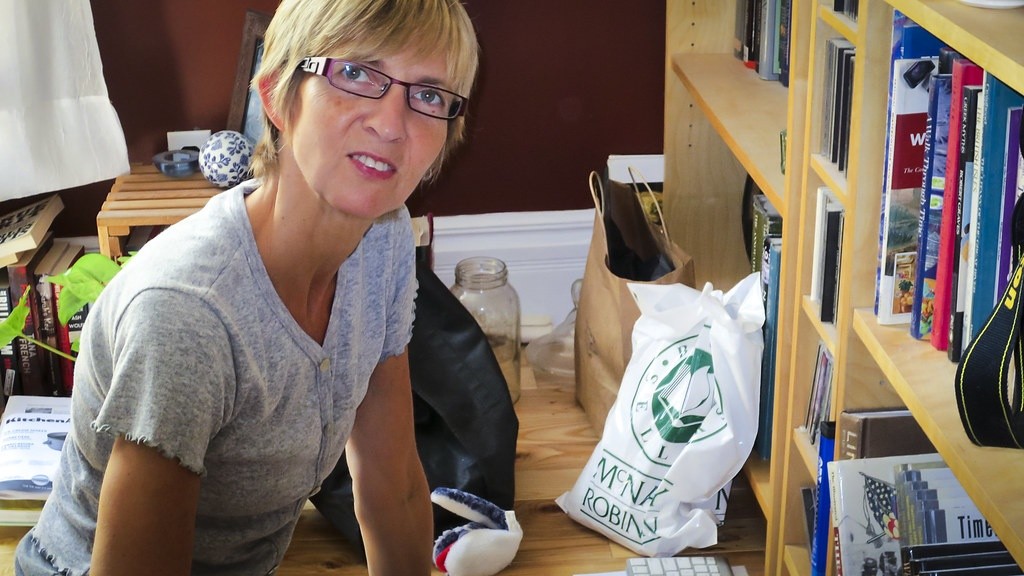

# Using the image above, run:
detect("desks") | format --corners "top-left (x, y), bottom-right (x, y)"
top-left (95, 165), bottom-right (245, 261)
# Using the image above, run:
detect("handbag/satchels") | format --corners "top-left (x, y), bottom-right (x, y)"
top-left (556, 271), bottom-right (765, 556)
top-left (575, 168), bottom-right (697, 440)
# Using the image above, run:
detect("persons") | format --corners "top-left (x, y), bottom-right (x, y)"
top-left (14, 0), bottom-right (480, 576)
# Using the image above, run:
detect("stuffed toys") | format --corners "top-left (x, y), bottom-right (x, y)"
top-left (430, 489), bottom-right (524, 576)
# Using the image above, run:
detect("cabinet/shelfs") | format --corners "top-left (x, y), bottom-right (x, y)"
top-left (663, 0), bottom-right (1024, 575)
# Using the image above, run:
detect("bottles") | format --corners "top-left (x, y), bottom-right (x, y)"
top-left (447, 255), bottom-right (522, 406)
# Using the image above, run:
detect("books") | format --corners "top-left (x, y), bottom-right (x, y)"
top-left (734, 0), bottom-right (1024, 576)
top-left (0, 194), bottom-right (103, 407)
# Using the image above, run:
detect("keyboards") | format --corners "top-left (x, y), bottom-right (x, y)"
top-left (626, 557), bottom-right (733, 576)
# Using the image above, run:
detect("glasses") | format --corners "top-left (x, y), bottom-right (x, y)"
top-left (298, 57), bottom-right (469, 120)
